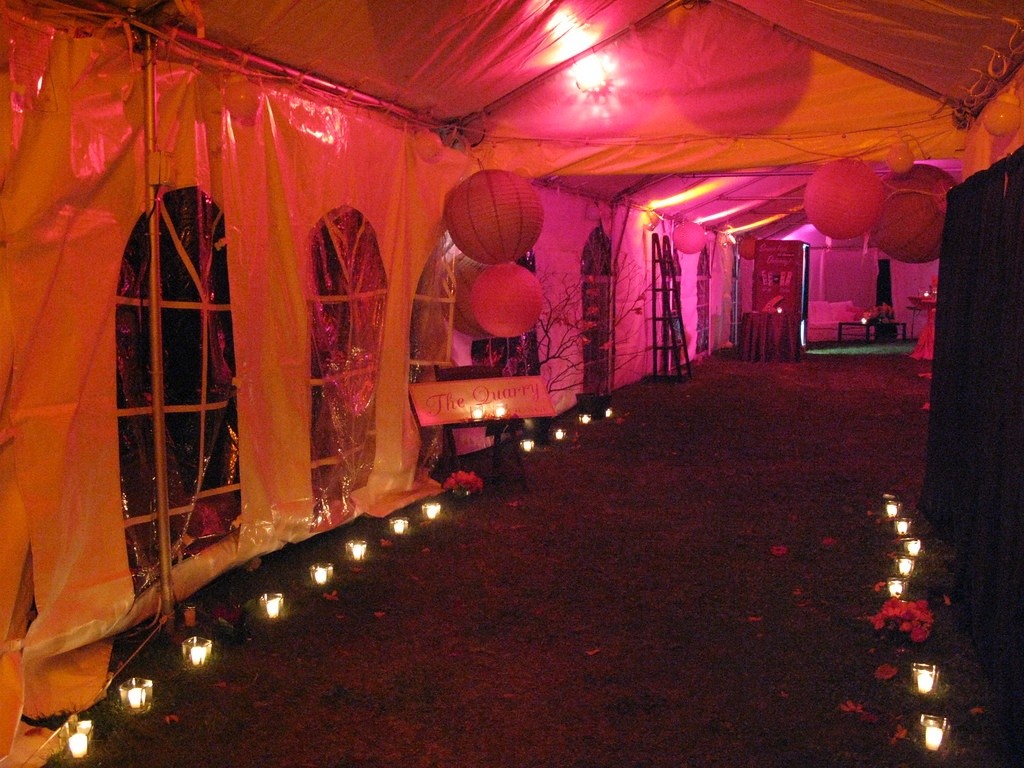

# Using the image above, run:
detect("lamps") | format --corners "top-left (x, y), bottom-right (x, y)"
top-left (982, 83), bottom-right (1021, 139)
top-left (886, 130), bottom-right (926, 175)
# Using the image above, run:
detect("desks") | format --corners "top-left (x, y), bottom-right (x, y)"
top-left (837, 322), bottom-right (906, 344)
top-left (740, 312), bottom-right (800, 363)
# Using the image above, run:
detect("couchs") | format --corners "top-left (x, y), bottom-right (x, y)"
top-left (806, 301), bottom-right (874, 349)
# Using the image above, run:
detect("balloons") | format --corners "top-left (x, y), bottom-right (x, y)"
top-left (739, 236), bottom-right (759, 260)
top-left (416, 133), bottom-right (444, 164)
top-left (887, 147), bottom-right (913, 174)
top-left (673, 222), bottom-right (705, 254)
top-left (982, 101), bottom-right (1021, 137)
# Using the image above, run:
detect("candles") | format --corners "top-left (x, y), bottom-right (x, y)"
top-left (183, 607), bottom-right (196, 628)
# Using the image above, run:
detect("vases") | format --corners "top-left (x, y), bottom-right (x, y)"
top-left (576, 393), bottom-right (611, 419)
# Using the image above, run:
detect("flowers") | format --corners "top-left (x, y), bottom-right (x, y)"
top-left (442, 470), bottom-right (485, 494)
top-left (864, 302), bottom-right (896, 318)
top-left (868, 596), bottom-right (935, 644)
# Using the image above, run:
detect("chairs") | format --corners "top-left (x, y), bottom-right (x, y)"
top-left (432, 363), bottom-right (527, 487)
